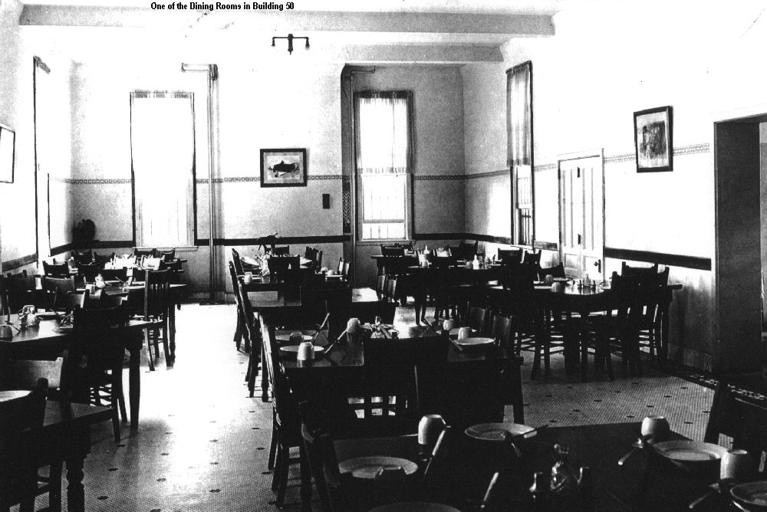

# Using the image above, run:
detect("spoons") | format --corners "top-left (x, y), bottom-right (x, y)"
top-left (513, 423), bottom-right (548, 441)
top-left (617, 433), bottom-right (652, 468)
top-left (686, 481), bottom-right (722, 510)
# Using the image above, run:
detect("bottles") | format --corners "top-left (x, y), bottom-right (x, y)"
top-left (578, 273), bottom-right (596, 289)
top-left (440, 330), bottom-right (450, 345)
top-left (526, 471), bottom-right (545, 512)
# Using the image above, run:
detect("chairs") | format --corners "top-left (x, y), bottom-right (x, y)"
top-left (228, 246), bottom-right (766, 510)
top-left (1, 245), bottom-right (188, 512)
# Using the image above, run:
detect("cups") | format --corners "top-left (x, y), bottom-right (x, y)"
top-left (0, 325), bottom-right (13, 339)
top-left (346, 318), bottom-right (361, 337)
top-left (85, 284), bottom-right (95, 294)
top-left (289, 332), bottom-right (303, 342)
top-left (399, 459), bottom-right (434, 504)
top-left (297, 341), bottom-right (315, 360)
top-left (418, 414), bottom-right (446, 445)
top-left (719, 447), bottom-right (754, 483)
top-left (443, 319), bottom-right (455, 330)
top-left (244, 272), bottom-right (252, 283)
top-left (639, 414), bottom-right (668, 441)
top-left (457, 326), bottom-right (471, 341)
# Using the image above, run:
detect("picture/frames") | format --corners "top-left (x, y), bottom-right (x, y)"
top-left (0, 125), bottom-right (16, 184)
top-left (259, 147), bottom-right (308, 187)
top-left (634, 106), bottom-right (674, 173)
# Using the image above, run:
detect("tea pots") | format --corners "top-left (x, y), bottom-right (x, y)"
top-left (546, 442), bottom-right (590, 511)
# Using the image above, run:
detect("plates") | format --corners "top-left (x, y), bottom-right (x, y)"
top-left (276, 335), bottom-right (313, 341)
top-left (337, 455), bottom-right (416, 480)
top-left (728, 480), bottom-right (767, 508)
top-left (652, 438), bottom-right (729, 463)
top-left (436, 328), bottom-right (476, 336)
top-left (532, 273), bottom-right (570, 293)
top-left (59, 326), bottom-right (72, 332)
top-left (600, 286), bottom-right (611, 290)
top-left (374, 502), bottom-right (465, 511)
top-left (463, 422), bottom-right (538, 442)
top-left (241, 277), bottom-right (260, 281)
top-left (456, 337), bottom-right (497, 347)
top-left (279, 344), bottom-right (324, 353)
top-left (362, 324), bottom-right (392, 331)
top-left (38, 311), bottom-right (66, 318)
top-left (0, 389), bottom-right (34, 403)
